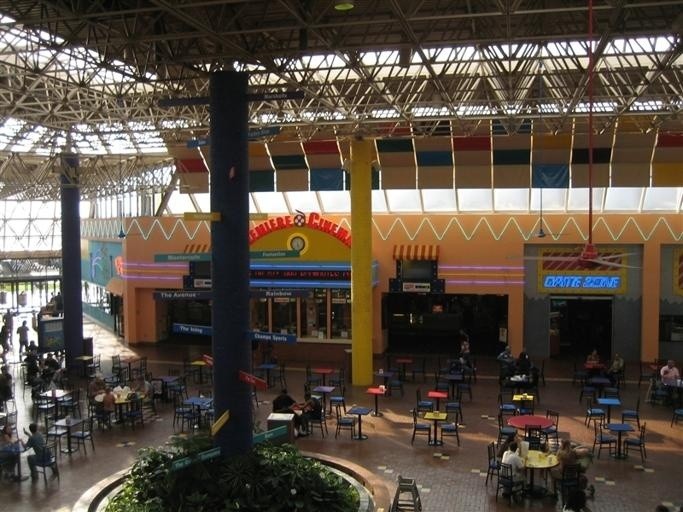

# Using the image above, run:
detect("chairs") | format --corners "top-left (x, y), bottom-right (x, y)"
top-left (573, 357), bottom-right (683, 462)
top-left (486, 356), bottom-right (595, 507)
top-left (249, 356), bottom-right (479, 448)
top-left (1, 354), bottom-right (214, 485)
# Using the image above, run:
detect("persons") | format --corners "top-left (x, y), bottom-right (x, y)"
top-left (1, 278), bottom-right (156, 482)
top-left (582, 348), bottom-right (599, 364)
top-left (493, 435), bottom-right (508, 465)
top-left (448, 340), bottom-right (475, 375)
top-left (515, 350), bottom-right (538, 382)
top-left (606, 350), bottom-right (625, 387)
top-left (500, 441), bottom-right (529, 505)
top-left (269, 387), bottom-right (304, 439)
top-left (293, 391), bottom-right (322, 436)
top-left (495, 343), bottom-right (515, 384)
top-left (658, 358), bottom-right (682, 409)
top-left (546, 439), bottom-right (590, 501)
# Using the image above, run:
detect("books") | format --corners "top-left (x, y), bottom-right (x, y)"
top-left (518, 441), bottom-right (529, 459)
